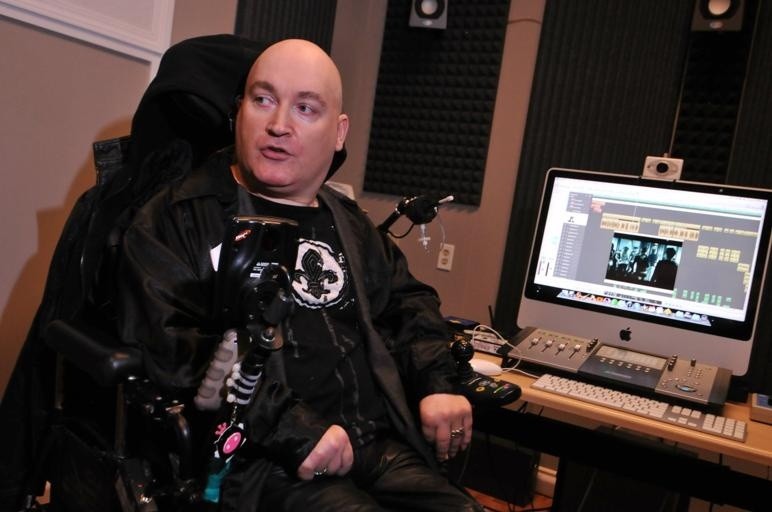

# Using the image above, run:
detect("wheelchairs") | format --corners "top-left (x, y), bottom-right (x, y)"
top-left (33, 302), bottom-right (527, 512)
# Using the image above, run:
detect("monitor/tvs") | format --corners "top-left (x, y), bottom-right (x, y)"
top-left (517, 167), bottom-right (772, 377)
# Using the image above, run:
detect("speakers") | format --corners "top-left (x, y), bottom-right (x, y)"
top-left (409, 0), bottom-right (448, 29)
top-left (691, 0), bottom-right (744, 32)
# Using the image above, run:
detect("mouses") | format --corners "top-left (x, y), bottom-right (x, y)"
top-left (470, 358), bottom-right (502, 376)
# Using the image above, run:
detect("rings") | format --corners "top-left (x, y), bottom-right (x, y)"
top-left (450, 427), bottom-right (464, 439)
top-left (314, 467), bottom-right (327, 476)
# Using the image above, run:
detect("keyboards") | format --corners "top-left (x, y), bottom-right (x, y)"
top-left (529, 373), bottom-right (747, 443)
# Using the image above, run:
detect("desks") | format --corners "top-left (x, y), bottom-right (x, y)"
top-left (451, 317), bottom-right (772, 479)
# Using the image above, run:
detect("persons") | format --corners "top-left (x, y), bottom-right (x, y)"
top-left (113, 36), bottom-right (493, 512)
top-left (606, 234), bottom-right (678, 292)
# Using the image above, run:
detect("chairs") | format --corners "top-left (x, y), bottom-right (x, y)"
top-left (40, 34), bottom-right (487, 510)
